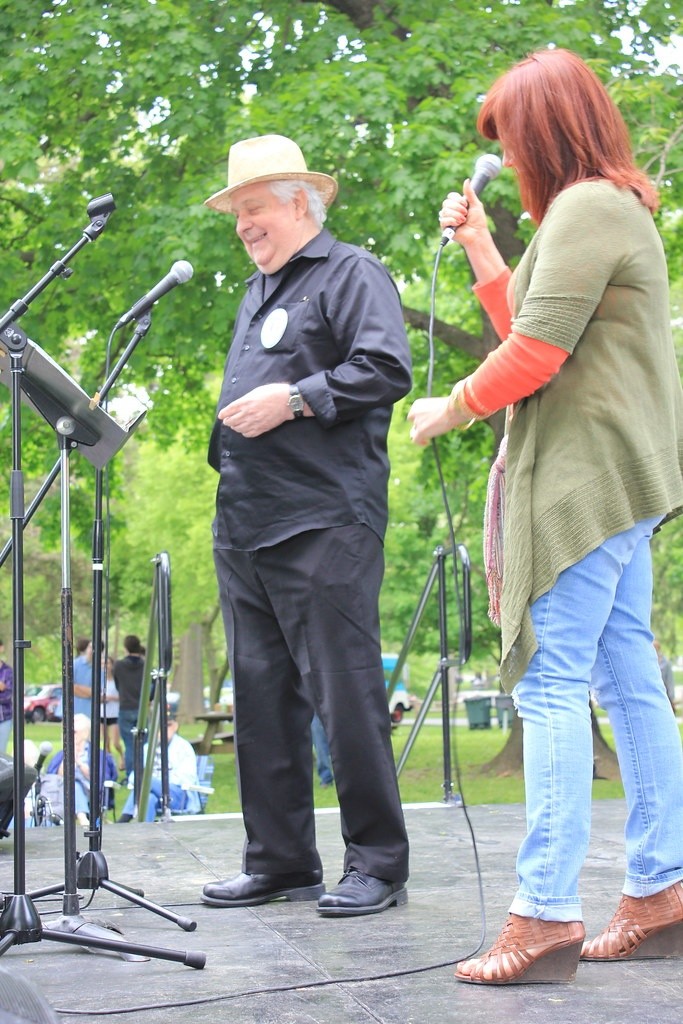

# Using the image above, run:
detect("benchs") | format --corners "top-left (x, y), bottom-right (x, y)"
top-left (188, 732), bottom-right (233, 749)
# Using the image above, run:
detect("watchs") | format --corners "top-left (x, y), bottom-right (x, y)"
top-left (287, 384), bottom-right (304, 419)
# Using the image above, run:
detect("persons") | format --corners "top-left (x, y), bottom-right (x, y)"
top-left (0, 635), bottom-right (200, 825)
top-left (201, 134), bottom-right (413, 916)
top-left (307, 712), bottom-right (333, 788)
top-left (408, 50), bottom-right (683, 983)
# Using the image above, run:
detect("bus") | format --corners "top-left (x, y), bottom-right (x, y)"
top-left (377, 652), bottom-right (412, 723)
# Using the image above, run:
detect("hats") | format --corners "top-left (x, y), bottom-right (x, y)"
top-left (164, 692), bottom-right (180, 721)
top-left (203, 133), bottom-right (339, 213)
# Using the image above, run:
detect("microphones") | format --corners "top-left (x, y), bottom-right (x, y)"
top-left (114, 260), bottom-right (194, 331)
top-left (440, 153), bottom-right (503, 246)
top-left (33, 741), bottom-right (53, 774)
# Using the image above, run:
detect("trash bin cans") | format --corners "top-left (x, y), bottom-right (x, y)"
top-left (463, 697), bottom-right (491, 729)
top-left (496, 695), bottom-right (515, 728)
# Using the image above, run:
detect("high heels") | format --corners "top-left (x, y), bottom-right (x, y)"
top-left (453, 911), bottom-right (583, 984)
top-left (579, 881), bottom-right (682, 960)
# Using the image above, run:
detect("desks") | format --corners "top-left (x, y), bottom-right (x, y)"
top-left (195, 713), bottom-right (232, 755)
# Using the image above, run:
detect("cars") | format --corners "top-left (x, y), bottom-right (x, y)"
top-left (21, 684), bottom-right (63, 724)
top-left (165, 694), bottom-right (211, 723)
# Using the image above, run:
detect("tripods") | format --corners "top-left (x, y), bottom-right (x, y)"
top-left (0, 311), bottom-right (198, 931)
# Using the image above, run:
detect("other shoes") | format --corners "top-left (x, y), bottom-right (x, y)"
top-left (118, 751), bottom-right (126, 770)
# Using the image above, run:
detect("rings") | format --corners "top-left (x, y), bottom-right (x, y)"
top-left (414, 425), bottom-right (417, 431)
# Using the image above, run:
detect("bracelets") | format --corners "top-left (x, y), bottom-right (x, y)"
top-left (446, 374), bottom-right (499, 431)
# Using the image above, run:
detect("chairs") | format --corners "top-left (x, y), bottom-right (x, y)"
top-left (104, 755), bottom-right (216, 813)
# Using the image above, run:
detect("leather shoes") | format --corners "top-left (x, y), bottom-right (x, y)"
top-left (315, 867), bottom-right (408, 914)
top-left (200, 867), bottom-right (325, 908)
